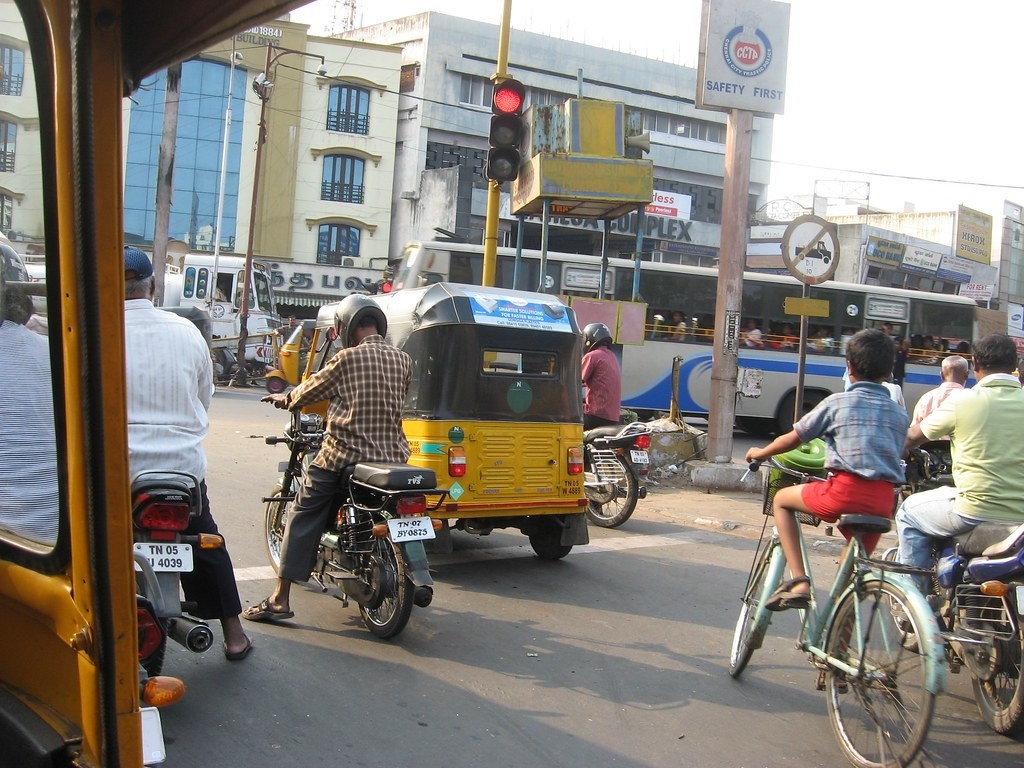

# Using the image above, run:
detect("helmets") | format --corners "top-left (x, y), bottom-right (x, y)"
top-left (582, 323), bottom-right (613, 356)
top-left (0, 242), bottom-right (35, 324)
top-left (333, 293), bottom-right (388, 348)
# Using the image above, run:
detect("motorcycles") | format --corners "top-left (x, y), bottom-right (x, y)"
top-left (1, 0), bottom-right (331, 768)
top-left (265, 281), bottom-right (590, 559)
top-left (585, 422), bottom-right (660, 528)
top-left (886, 447), bottom-right (1024, 734)
top-left (128, 471), bottom-right (223, 677)
top-left (261, 401), bottom-right (451, 639)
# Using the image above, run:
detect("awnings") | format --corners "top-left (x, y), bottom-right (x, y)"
top-left (259, 295), bottom-right (338, 307)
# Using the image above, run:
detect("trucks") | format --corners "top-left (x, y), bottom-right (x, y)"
top-left (17, 255), bottom-right (282, 384)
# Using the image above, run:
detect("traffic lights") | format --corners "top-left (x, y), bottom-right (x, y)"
top-left (381, 280), bottom-right (391, 293)
top-left (486, 78), bottom-right (526, 182)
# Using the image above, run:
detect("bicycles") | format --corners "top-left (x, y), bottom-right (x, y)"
top-left (728, 459), bottom-right (947, 768)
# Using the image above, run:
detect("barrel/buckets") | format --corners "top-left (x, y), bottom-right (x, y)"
top-left (767, 434), bottom-right (828, 500)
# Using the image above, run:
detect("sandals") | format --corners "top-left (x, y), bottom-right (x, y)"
top-left (764, 574), bottom-right (813, 610)
top-left (890, 603), bottom-right (909, 621)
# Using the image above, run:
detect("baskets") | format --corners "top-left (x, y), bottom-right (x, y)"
top-left (762, 466), bottom-right (823, 527)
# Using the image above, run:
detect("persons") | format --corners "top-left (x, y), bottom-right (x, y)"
top-left (910, 355), bottom-right (970, 442)
top-left (663, 311), bottom-right (686, 341)
top-left (740, 319), bottom-right (853, 356)
top-left (241, 293), bottom-right (413, 620)
top-left (0, 244), bottom-right (60, 547)
top-left (890, 333), bottom-right (1024, 621)
top-left (581, 322), bottom-right (622, 432)
top-left (124, 245), bottom-right (254, 659)
top-left (746, 328), bottom-right (909, 694)
top-left (883, 324), bottom-right (969, 365)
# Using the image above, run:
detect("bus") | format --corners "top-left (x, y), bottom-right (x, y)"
top-left (391, 239), bottom-right (978, 436)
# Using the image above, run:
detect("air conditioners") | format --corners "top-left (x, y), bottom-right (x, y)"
top-left (341, 255), bottom-right (363, 268)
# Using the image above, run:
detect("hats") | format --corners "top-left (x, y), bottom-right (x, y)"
top-left (123, 244), bottom-right (152, 283)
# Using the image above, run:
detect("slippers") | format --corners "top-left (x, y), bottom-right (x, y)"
top-left (222, 627), bottom-right (252, 660)
top-left (243, 599), bottom-right (294, 621)
